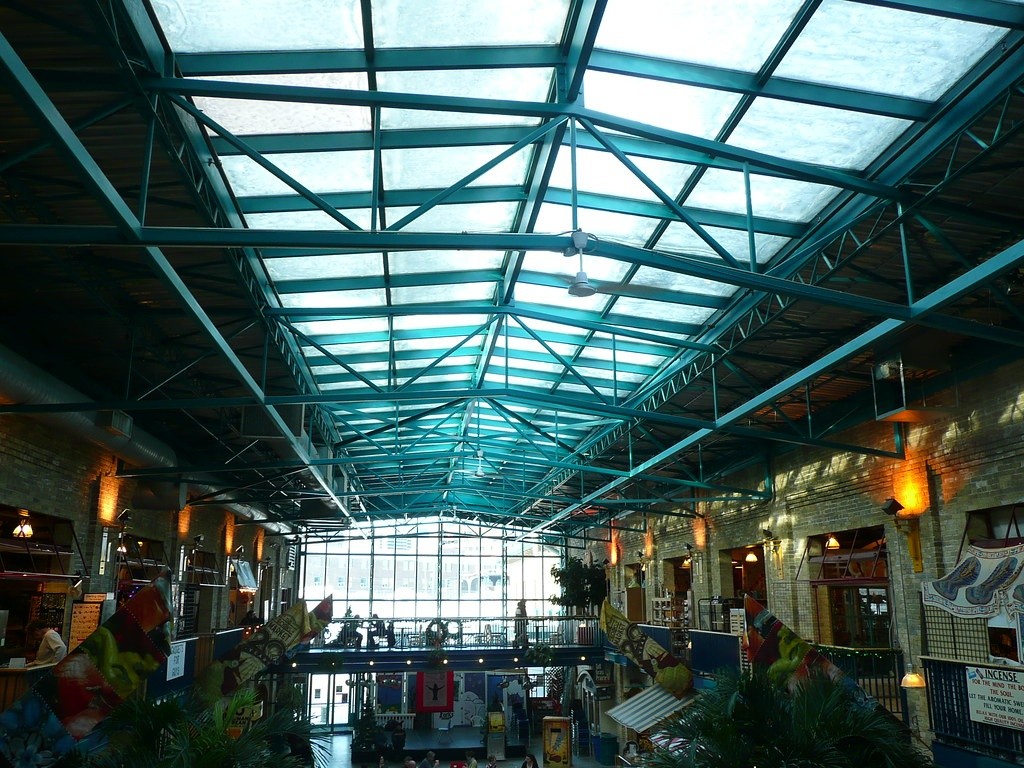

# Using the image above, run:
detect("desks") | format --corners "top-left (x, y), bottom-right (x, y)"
top-left (453, 627), bottom-right (469, 648)
top-left (361, 626), bottom-right (376, 648)
top-left (395, 627), bottom-right (411, 649)
top-left (533, 625), bottom-right (547, 645)
top-left (0, 661), bottom-right (62, 713)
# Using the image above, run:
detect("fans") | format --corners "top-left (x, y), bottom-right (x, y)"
top-left (453, 450), bottom-right (498, 477)
top-left (522, 231), bottom-right (671, 297)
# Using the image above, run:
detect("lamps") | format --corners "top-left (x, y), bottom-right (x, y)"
top-left (882, 499), bottom-right (904, 517)
top-left (684, 542), bottom-right (693, 552)
top-left (192, 533), bottom-right (206, 545)
top-left (264, 556), bottom-right (270, 561)
top-left (602, 558), bottom-right (609, 565)
top-left (636, 551), bottom-right (643, 558)
top-left (893, 517), bottom-right (926, 689)
top-left (235, 545), bottom-right (245, 554)
top-left (71, 573), bottom-right (82, 588)
top-left (763, 529), bottom-right (772, 540)
top-left (117, 508), bottom-right (131, 525)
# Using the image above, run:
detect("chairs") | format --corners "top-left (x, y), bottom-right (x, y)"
top-left (570, 698), bottom-right (590, 758)
top-left (510, 701), bottom-right (530, 739)
top-left (436, 718), bottom-right (454, 743)
top-left (339, 624), bottom-right (565, 649)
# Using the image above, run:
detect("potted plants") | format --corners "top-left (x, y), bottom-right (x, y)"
top-left (550, 556), bottom-right (611, 645)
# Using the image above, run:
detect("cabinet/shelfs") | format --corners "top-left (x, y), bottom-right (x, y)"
top-left (698, 598), bottom-right (739, 634)
top-left (650, 597), bottom-right (685, 627)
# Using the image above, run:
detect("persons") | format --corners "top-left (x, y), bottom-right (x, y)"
top-left (348, 615), bottom-right (363, 647)
top-left (29, 620), bottom-right (67, 666)
top-left (368, 614), bottom-right (386, 646)
top-left (361, 750), bottom-right (540, 768)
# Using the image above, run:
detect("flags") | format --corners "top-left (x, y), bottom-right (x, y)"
top-left (741, 594), bottom-right (877, 729)
top-left (36, 579), bottom-right (173, 744)
top-left (599, 601), bottom-right (669, 674)
top-left (206, 601), bottom-right (317, 698)
top-left (270, 595), bottom-right (333, 673)
